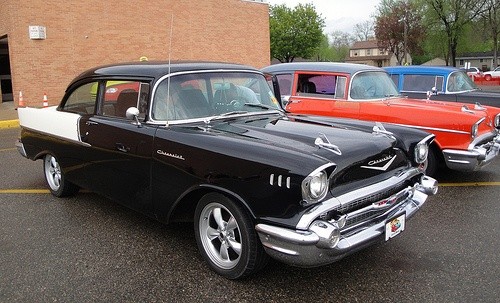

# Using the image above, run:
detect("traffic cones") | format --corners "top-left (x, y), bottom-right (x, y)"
top-left (42, 93), bottom-right (49, 107)
top-left (15, 89), bottom-right (26, 109)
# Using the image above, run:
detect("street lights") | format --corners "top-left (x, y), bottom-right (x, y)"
top-left (398, 20), bottom-right (407, 63)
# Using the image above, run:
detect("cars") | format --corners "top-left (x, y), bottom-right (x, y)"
top-left (243, 62), bottom-right (500, 178)
top-left (483, 67), bottom-right (500, 81)
top-left (15, 61), bottom-right (440, 281)
top-left (330, 66), bottom-right (500, 108)
top-left (89, 57), bottom-right (199, 104)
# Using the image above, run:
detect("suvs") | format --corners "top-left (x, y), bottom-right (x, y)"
top-left (460, 67), bottom-right (484, 81)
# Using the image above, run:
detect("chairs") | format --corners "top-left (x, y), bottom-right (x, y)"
top-left (86, 104), bottom-right (115, 116)
top-left (277, 79), bottom-right (291, 96)
top-left (115, 89), bottom-right (149, 118)
top-left (177, 89), bottom-right (210, 119)
top-left (303, 82), bottom-right (316, 94)
